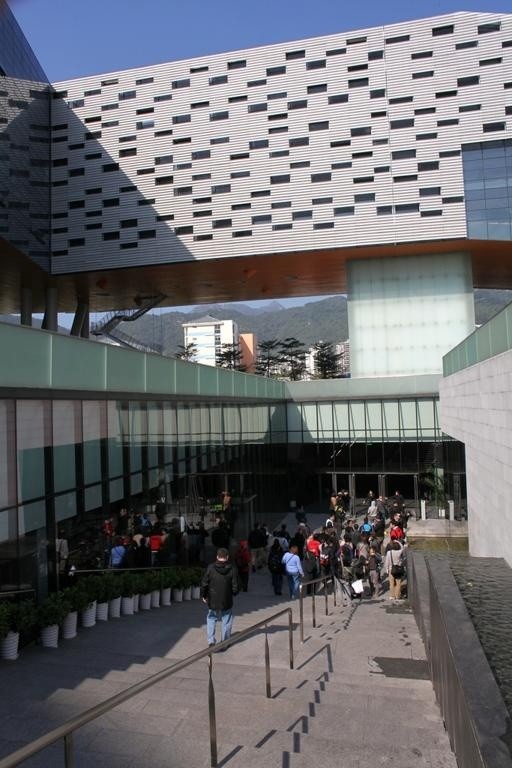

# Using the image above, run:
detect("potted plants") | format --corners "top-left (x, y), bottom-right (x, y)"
top-left (1, 565), bottom-right (207, 661)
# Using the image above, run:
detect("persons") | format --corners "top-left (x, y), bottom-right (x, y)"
top-left (248, 521), bottom-right (268, 573)
top-left (257, 487), bottom-right (413, 601)
top-left (56, 488), bottom-right (235, 574)
top-left (236, 540), bottom-right (252, 593)
top-left (200, 547), bottom-right (234, 653)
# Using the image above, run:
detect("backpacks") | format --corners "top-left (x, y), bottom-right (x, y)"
top-left (269, 547), bottom-right (282, 572)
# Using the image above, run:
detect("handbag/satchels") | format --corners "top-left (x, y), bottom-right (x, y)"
top-left (351, 579), bottom-right (364, 594)
top-left (341, 566), bottom-right (354, 582)
top-left (391, 564), bottom-right (406, 579)
